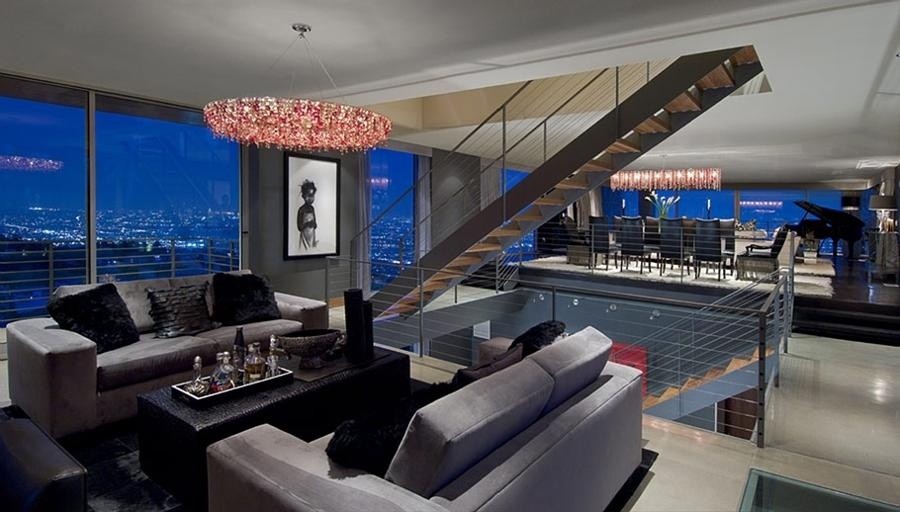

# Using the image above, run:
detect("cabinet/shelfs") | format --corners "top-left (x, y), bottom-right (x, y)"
top-left (867, 232), bottom-right (899, 283)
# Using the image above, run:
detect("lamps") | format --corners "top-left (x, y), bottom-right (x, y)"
top-left (869, 194), bottom-right (898, 231)
top-left (610, 154), bottom-right (722, 192)
top-left (200, 23), bottom-right (393, 155)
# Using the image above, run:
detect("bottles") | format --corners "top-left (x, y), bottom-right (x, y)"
top-left (185, 356), bottom-right (209, 395)
top-left (266, 335), bottom-right (279, 376)
top-left (243, 342), bottom-right (266, 381)
top-left (224, 351), bottom-right (234, 375)
top-left (209, 352), bottom-right (235, 393)
top-left (232, 325), bottom-right (247, 384)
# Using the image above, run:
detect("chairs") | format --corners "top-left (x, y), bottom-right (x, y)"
top-left (556, 212), bottom-right (738, 282)
top-left (733, 223), bottom-right (789, 284)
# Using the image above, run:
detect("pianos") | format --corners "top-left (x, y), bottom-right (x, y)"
top-left (782, 200), bottom-right (866, 268)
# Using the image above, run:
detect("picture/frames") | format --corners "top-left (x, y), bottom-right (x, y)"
top-left (281, 150), bottom-right (342, 262)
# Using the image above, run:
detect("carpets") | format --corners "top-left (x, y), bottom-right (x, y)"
top-left (508, 252), bottom-right (837, 300)
top-left (0, 377), bottom-right (660, 511)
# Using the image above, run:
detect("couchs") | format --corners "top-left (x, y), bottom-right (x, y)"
top-left (5, 269), bottom-right (329, 439)
top-left (206, 326), bottom-right (644, 512)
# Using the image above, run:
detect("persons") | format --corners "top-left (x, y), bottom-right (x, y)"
top-left (295, 177), bottom-right (320, 251)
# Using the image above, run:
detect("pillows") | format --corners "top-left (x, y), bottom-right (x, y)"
top-left (211, 272), bottom-right (283, 325)
top-left (143, 281), bottom-right (223, 338)
top-left (324, 380), bottom-right (462, 480)
top-left (46, 283), bottom-right (138, 353)
top-left (452, 342), bottom-right (523, 387)
top-left (507, 320), bottom-right (566, 357)
